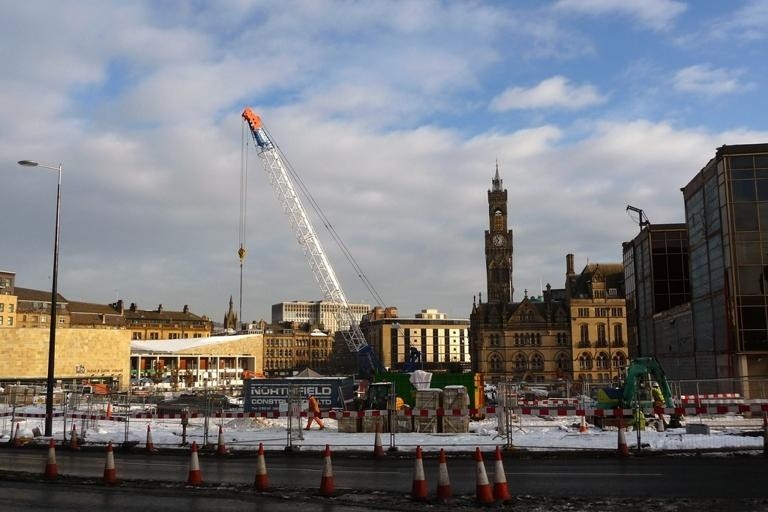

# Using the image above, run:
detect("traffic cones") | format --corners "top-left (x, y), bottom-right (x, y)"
top-left (101, 440), bottom-right (118, 482)
top-left (374, 422), bottom-right (385, 456)
top-left (13, 421), bottom-right (21, 447)
top-left (145, 424), bottom-right (159, 453)
top-left (615, 414), bottom-right (631, 457)
top-left (762, 410), bottom-right (768, 426)
top-left (254, 441), bottom-right (270, 491)
top-left (579, 413), bottom-right (586, 432)
top-left (318, 442), bottom-right (335, 494)
top-left (186, 439), bottom-right (205, 485)
top-left (216, 424), bottom-right (229, 455)
top-left (43, 438), bottom-right (58, 480)
top-left (68, 424), bottom-right (80, 450)
top-left (105, 401), bottom-right (112, 417)
top-left (411, 442), bottom-right (510, 503)
top-left (657, 413), bottom-right (665, 432)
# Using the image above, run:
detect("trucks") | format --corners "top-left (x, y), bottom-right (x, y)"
top-left (75, 384), bottom-right (107, 395)
top-left (362, 370), bottom-right (485, 422)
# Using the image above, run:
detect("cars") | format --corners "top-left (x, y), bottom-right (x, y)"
top-left (153, 392), bottom-right (244, 413)
top-left (483, 382), bottom-right (565, 400)
top-left (33, 390), bottom-right (71, 405)
top-left (133, 377), bottom-right (154, 385)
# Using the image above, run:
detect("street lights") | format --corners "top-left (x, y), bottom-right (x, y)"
top-left (17, 157), bottom-right (64, 439)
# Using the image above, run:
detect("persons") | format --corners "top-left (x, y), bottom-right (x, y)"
top-left (302, 393), bottom-right (325, 430)
top-left (640, 381), bottom-right (666, 424)
top-left (634, 405), bottom-right (646, 431)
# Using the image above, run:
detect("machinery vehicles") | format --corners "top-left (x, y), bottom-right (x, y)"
top-left (592, 356), bottom-right (682, 429)
